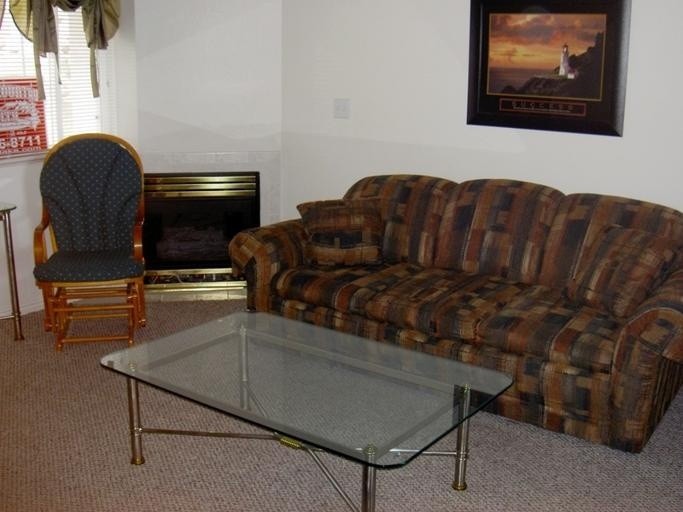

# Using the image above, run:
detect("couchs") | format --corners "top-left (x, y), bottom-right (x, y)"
top-left (226, 173), bottom-right (683, 453)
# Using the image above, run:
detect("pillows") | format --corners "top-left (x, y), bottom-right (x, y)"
top-left (294, 196), bottom-right (384, 269)
top-left (561, 208), bottom-right (675, 321)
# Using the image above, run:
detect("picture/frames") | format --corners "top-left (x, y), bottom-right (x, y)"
top-left (465, 1), bottom-right (632, 138)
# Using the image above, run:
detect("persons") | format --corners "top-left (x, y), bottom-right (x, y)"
top-left (559, 45), bottom-right (569, 76)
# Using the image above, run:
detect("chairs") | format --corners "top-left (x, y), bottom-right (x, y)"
top-left (30, 131), bottom-right (149, 348)
